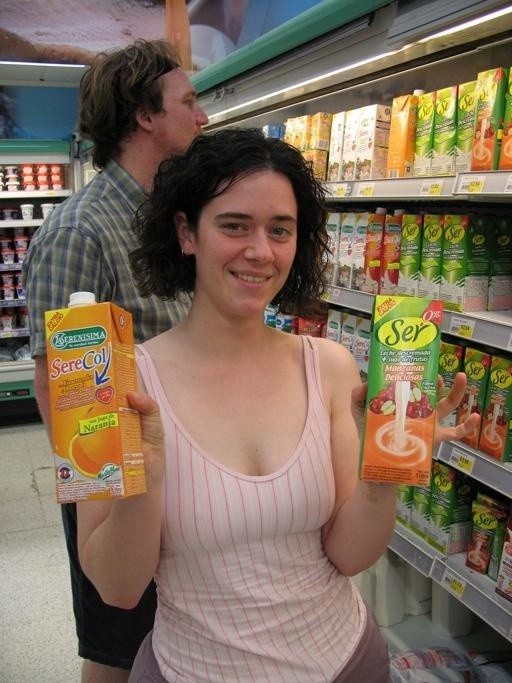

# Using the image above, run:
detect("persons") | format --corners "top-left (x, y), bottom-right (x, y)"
top-left (20, 36), bottom-right (210, 682)
top-left (73, 126), bottom-right (484, 683)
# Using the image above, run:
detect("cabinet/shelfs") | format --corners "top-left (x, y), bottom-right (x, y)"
top-left (190, 2), bottom-right (511, 682)
top-left (0, 137), bottom-right (83, 401)
